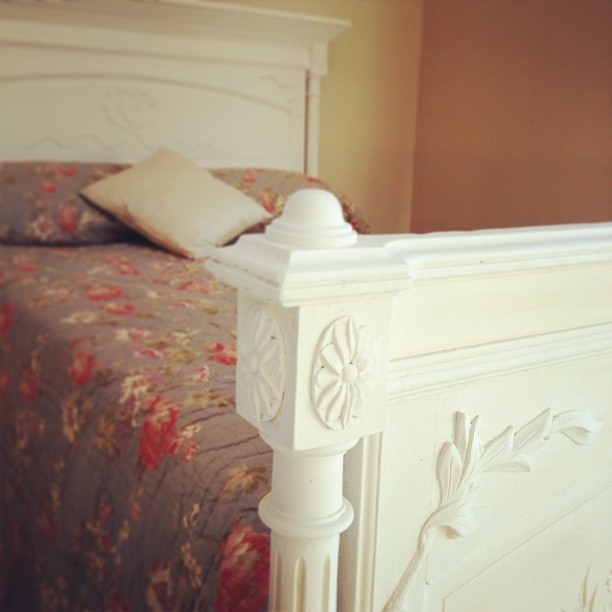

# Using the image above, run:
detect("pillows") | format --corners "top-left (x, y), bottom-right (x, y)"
top-left (79, 148), bottom-right (271, 256)
top-left (1, 157), bottom-right (132, 245)
top-left (204, 164), bottom-right (355, 233)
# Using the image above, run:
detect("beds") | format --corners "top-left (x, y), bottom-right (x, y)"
top-left (0, 6), bottom-right (611, 612)
